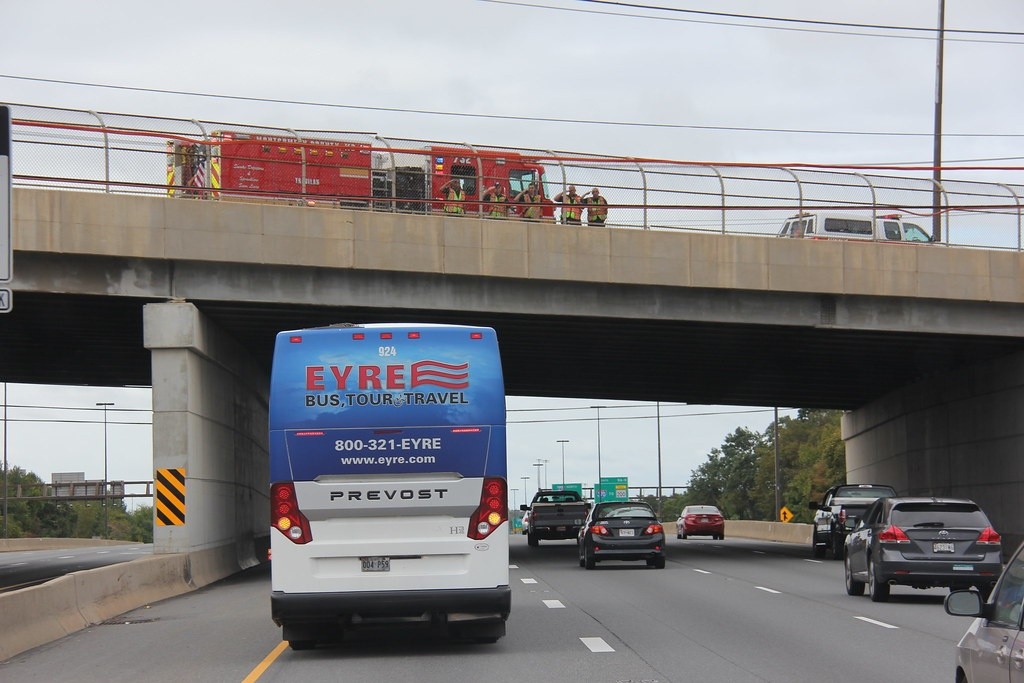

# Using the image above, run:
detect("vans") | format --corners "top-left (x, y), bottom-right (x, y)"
top-left (777, 213), bottom-right (936, 246)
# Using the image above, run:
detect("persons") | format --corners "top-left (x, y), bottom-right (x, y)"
top-left (580, 188), bottom-right (608, 227)
top-left (483, 182), bottom-right (510, 217)
top-left (513, 184), bottom-right (543, 218)
top-left (440, 178), bottom-right (466, 217)
top-left (555, 186), bottom-right (582, 225)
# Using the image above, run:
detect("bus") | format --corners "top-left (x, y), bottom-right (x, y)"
top-left (267, 322), bottom-right (512, 651)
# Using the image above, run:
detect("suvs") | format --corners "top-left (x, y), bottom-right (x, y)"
top-left (577, 500), bottom-right (666, 569)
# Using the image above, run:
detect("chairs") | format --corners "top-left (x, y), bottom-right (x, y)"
top-left (565, 497), bottom-right (573, 502)
top-left (540, 498), bottom-right (549, 502)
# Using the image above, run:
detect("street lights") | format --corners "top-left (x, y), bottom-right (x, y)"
top-left (590, 406), bottom-right (608, 502)
top-left (510, 488), bottom-right (520, 533)
top-left (533, 463), bottom-right (544, 491)
top-left (520, 476), bottom-right (530, 505)
top-left (96, 402), bottom-right (114, 540)
top-left (556, 440), bottom-right (570, 490)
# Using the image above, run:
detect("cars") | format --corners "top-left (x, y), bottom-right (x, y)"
top-left (843, 495), bottom-right (1004, 604)
top-left (521, 509), bottom-right (530, 535)
top-left (943, 537), bottom-right (1024, 683)
top-left (675, 504), bottom-right (725, 539)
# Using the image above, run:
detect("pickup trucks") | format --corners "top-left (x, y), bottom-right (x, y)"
top-left (519, 488), bottom-right (591, 547)
top-left (808, 483), bottom-right (898, 561)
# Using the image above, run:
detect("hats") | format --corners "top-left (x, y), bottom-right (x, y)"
top-left (494, 182), bottom-right (500, 186)
top-left (454, 178), bottom-right (460, 184)
top-left (568, 185), bottom-right (577, 190)
top-left (593, 188), bottom-right (598, 191)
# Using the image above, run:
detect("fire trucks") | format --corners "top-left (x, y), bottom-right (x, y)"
top-left (167, 129), bottom-right (555, 223)
